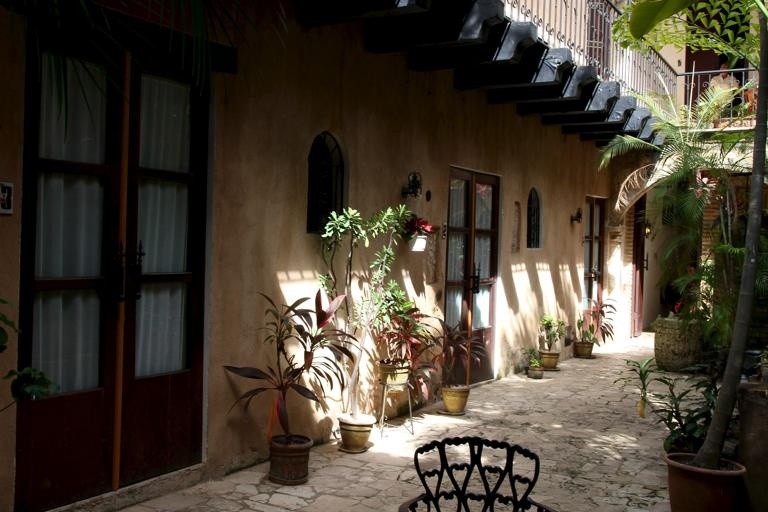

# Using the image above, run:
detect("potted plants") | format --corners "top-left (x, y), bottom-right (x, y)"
top-left (598, 67), bottom-right (766, 512)
top-left (217, 290), bottom-right (360, 486)
top-left (519, 293), bottom-right (619, 383)
top-left (432, 319), bottom-right (487, 418)
top-left (358, 288), bottom-right (435, 394)
top-left (311, 203), bottom-right (410, 453)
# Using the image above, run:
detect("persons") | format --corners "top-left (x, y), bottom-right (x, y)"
top-left (710, 64), bottom-right (742, 116)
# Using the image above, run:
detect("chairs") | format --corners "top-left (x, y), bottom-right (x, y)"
top-left (394, 435), bottom-right (559, 511)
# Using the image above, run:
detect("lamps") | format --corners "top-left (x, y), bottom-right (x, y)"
top-left (643, 216), bottom-right (653, 242)
top-left (401, 168), bottom-right (425, 203)
top-left (568, 205), bottom-right (584, 226)
